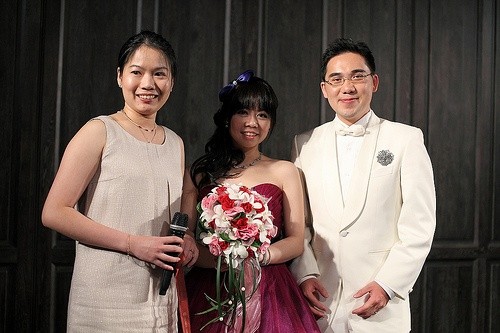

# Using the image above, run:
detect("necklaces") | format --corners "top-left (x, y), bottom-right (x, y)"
top-left (232, 150), bottom-right (264, 170)
top-left (119, 109), bottom-right (157, 143)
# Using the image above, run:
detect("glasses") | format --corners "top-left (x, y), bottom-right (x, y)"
top-left (324, 73), bottom-right (371, 87)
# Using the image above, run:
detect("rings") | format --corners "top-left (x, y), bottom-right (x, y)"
top-left (189, 248), bottom-right (196, 254)
top-left (372, 312), bottom-right (376, 316)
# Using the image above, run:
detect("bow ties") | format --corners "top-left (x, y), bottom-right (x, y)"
top-left (336, 123), bottom-right (365, 136)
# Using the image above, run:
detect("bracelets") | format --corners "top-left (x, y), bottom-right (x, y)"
top-left (266, 247), bottom-right (272, 265)
top-left (126, 233), bottom-right (133, 258)
top-left (261, 250), bottom-right (268, 266)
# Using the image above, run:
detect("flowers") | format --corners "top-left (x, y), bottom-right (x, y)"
top-left (197, 183), bottom-right (278, 333)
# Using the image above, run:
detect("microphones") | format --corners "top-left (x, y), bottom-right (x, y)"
top-left (159, 212), bottom-right (189, 295)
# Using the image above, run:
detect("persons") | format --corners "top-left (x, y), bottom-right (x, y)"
top-left (287, 37), bottom-right (437, 333)
top-left (40, 27), bottom-right (199, 333)
top-left (178, 68), bottom-right (323, 333)
